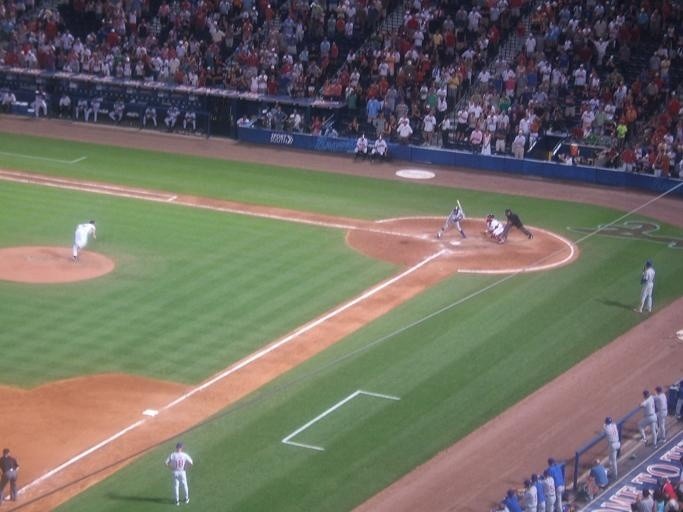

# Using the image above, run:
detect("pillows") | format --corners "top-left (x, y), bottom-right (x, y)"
top-left (504, 208), bottom-right (511, 216)
top-left (605, 417), bottom-right (613, 423)
top-left (453, 206), bottom-right (459, 212)
top-left (488, 214), bottom-right (494, 218)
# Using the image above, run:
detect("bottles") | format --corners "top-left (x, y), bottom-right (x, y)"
top-left (532, 474), bottom-right (537, 481)
top-left (646, 261), bottom-right (654, 267)
top-left (643, 390), bottom-right (650, 396)
top-left (656, 386), bottom-right (663, 392)
top-left (177, 443), bottom-right (183, 448)
top-left (507, 490), bottom-right (514, 498)
top-left (524, 479), bottom-right (531, 485)
top-left (548, 458), bottom-right (555, 464)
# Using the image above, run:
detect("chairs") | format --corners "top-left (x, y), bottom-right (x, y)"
top-left (176, 501), bottom-right (180, 506)
top-left (185, 498), bottom-right (189, 503)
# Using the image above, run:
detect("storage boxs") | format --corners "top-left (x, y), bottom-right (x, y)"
top-left (6, 87), bottom-right (210, 137)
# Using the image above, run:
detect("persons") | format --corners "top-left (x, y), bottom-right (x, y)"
top-left (1, 1), bottom-right (330, 136)
top-left (655, 387), bottom-right (667, 442)
top-left (499, 209), bottom-right (533, 243)
top-left (602, 417), bottom-right (620, 480)
top-left (631, 476), bottom-right (683, 511)
top-left (675, 381), bottom-right (682, 420)
top-left (637, 391), bottom-right (657, 448)
top-left (590, 459), bottom-right (608, 487)
top-left (502, 458), bottom-right (565, 511)
top-left (73, 220), bottom-right (96, 262)
top-left (1, 449), bottom-right (20, 501)
top-left (164, 442), bottom-right (193, 506)
top-left (560, 0), bottom-right (683, 179)
top-left (485, 214), bottom-right (504, 241)
top-left (324, 1), bottom-right (567, 161)
top-left (370, 134), bottom-right (387, 163)
top-left (354, 134), bottom-right (368, 162)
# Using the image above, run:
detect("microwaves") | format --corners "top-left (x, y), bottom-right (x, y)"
top-left (456, 199), bottom-right (463, 216)
